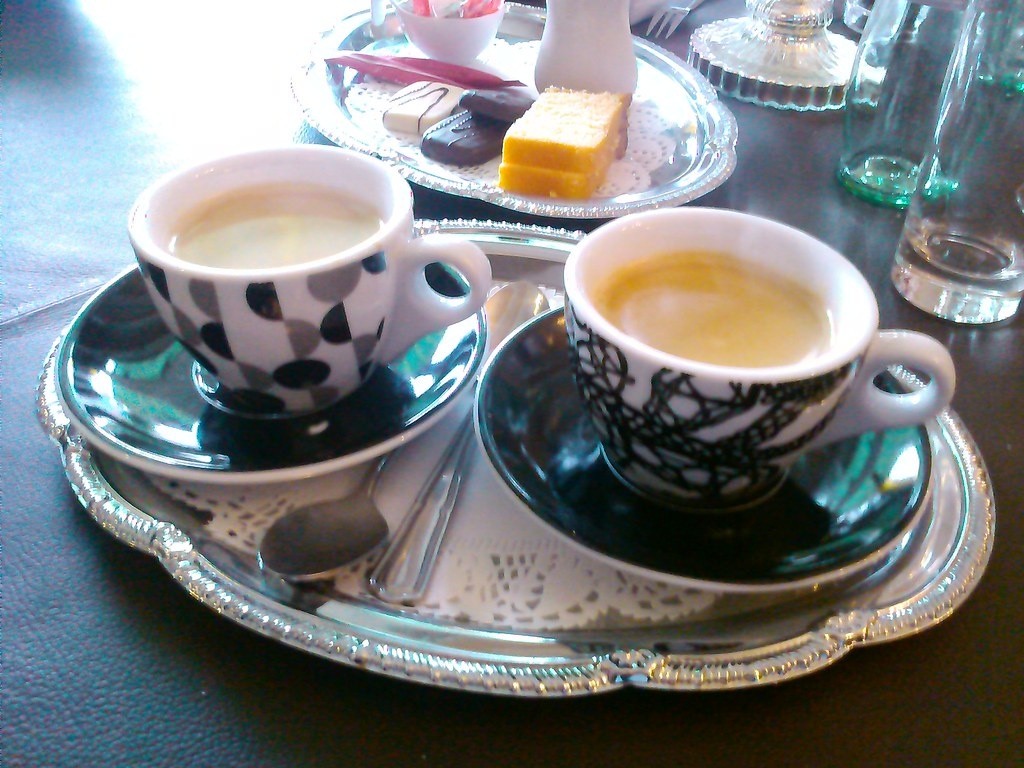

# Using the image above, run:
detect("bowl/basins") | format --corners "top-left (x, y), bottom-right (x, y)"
top-left (391, 0), bottom-right (504, 65)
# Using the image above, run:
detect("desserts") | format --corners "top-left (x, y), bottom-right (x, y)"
top-left (381, 81), bottom-right (538, 167)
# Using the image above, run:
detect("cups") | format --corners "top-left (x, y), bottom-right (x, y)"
top-left (890, 1), bottom-right (1024, 324)
top-left (126, 144), bottom-right (492, 415)
top-left (836, 0), bottom-right (1024, 211)
top-left (564, 207), bottom-right (956, 514)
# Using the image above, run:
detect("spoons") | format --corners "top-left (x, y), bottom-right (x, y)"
top-left (370, 281), bottom-right (549, 603)
top-left (257, 449), bottom-right (394, 577)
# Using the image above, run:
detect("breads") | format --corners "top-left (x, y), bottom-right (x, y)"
top-left (500, 87), bottom-right (629, 199)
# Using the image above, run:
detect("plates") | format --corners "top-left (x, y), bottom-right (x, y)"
top-left (474, 306), bottom-right (937, 592)
top-left (290, 2), bottom-right (738, 219)
top-left (35, 220), bottom-right (996, 697)
top-left (54, 262), bottom-right (488, 483)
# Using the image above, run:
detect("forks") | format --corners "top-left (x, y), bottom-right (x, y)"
top-left (646, 0), bottom-right (704, 39)
